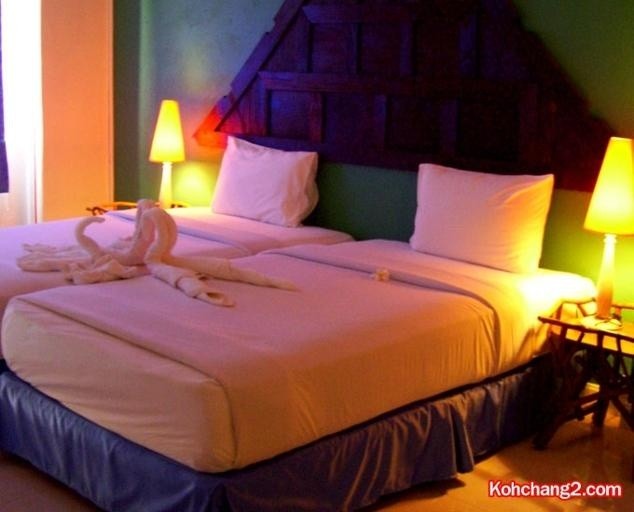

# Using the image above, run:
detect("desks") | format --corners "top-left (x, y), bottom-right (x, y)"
top-left (533, 292), bottom-right (633, 450)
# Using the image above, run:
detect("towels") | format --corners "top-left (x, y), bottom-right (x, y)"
top-left (17, 197), bottom-right (301, 307)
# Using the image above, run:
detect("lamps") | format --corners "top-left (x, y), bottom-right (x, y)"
top-left (581, 135), bottom-right (632, 334)
top-left (146, 100), bottom-right (186, 214)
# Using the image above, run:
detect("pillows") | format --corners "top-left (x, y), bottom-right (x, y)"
top-left (211, 136), bottom-right (317, 227)
